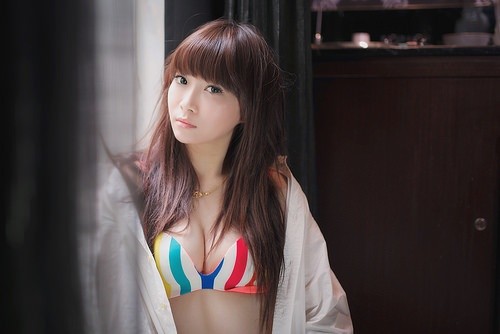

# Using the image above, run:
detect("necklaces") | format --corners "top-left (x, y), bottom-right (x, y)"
top-left (188, 175), bottom-right (231, 199)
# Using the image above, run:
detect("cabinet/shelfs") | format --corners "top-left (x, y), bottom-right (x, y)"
top-left (306, 0), bottom-right (500, 334)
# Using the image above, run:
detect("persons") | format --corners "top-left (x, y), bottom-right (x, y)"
top-left (92, 16), bottom-right (356, 334)
